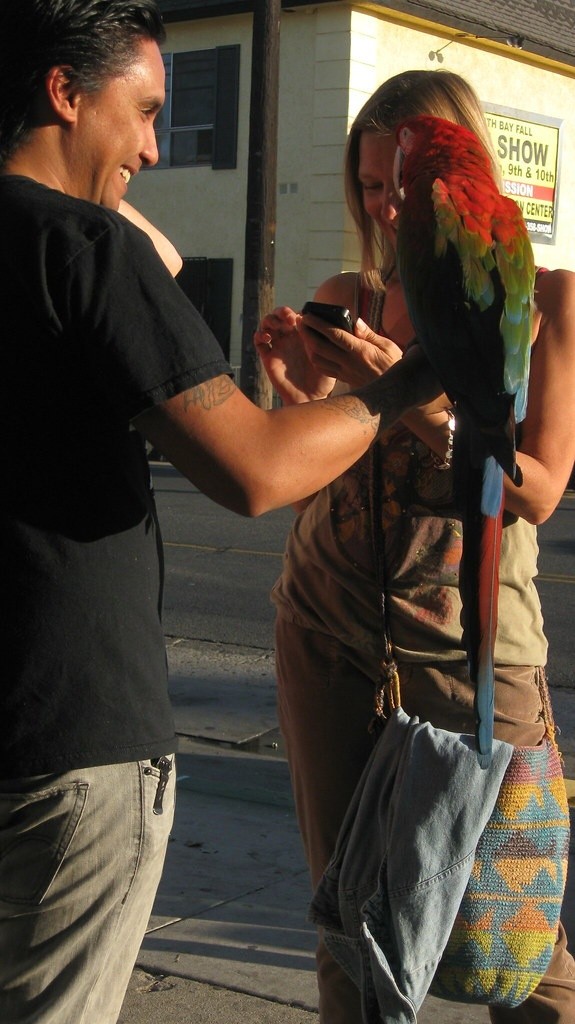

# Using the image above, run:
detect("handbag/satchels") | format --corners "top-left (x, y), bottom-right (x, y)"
top-left (355, 709), bottom-right (569, 1009)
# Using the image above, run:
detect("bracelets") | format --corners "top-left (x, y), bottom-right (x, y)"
top-left (431, 409), bottom-right (455, 470)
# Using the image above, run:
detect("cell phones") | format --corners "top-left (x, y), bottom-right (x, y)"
top-left (303, 301), bottom-right (354, 353)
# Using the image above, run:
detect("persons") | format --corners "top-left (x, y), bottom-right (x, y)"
top-left (0, 1), bottom-right (443, 1024)
top-left (255, 68), bottom-right (575, 1024)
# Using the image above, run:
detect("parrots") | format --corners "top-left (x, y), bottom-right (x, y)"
top-left (395, 114), bottom-right (536, 770)
